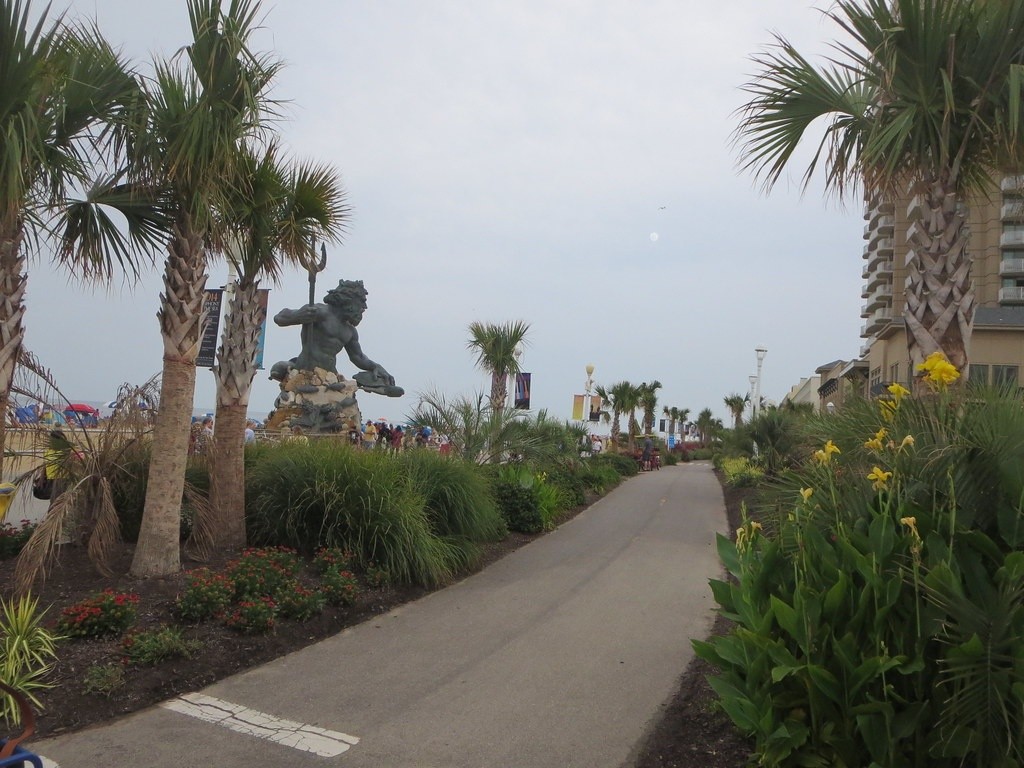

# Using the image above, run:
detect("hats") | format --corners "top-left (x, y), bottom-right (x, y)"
top-left (645, 434), bottom-right (650, 439)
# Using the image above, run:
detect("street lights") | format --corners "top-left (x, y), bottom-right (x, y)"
top-left (748, 372), bottom-right (758, 421)
top-left (580, 363), bottom-right (595, 456)
top-left (754, 345), bottom-right (768, 467)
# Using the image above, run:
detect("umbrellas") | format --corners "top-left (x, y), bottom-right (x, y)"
top-left (66, 404), bottom-right (95, 422)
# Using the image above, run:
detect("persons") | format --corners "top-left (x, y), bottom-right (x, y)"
top-left (590, 434), bottom-right (602, 454)
top-left (643, 434), bottom-right (652, 470)
top-left (192, 417), bottom-right (214, 454)
top-left (340, 418), bottom-right (453, 463)
top-left (91, 409), bottom-right (102, 428)
top-left (49, 422), bottom-right (67, 451)
top-left (274, 279), bottom-right (395, 386)
top-left (286, 425), bottom-right (309, 447)
top-left (244, 420), bottom-right (257, 448)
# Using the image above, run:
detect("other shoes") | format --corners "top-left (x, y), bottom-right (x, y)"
top-left (648, 468), bottom-right (651, 471)
top-left (643, 469), bottom-right (647, 470)
